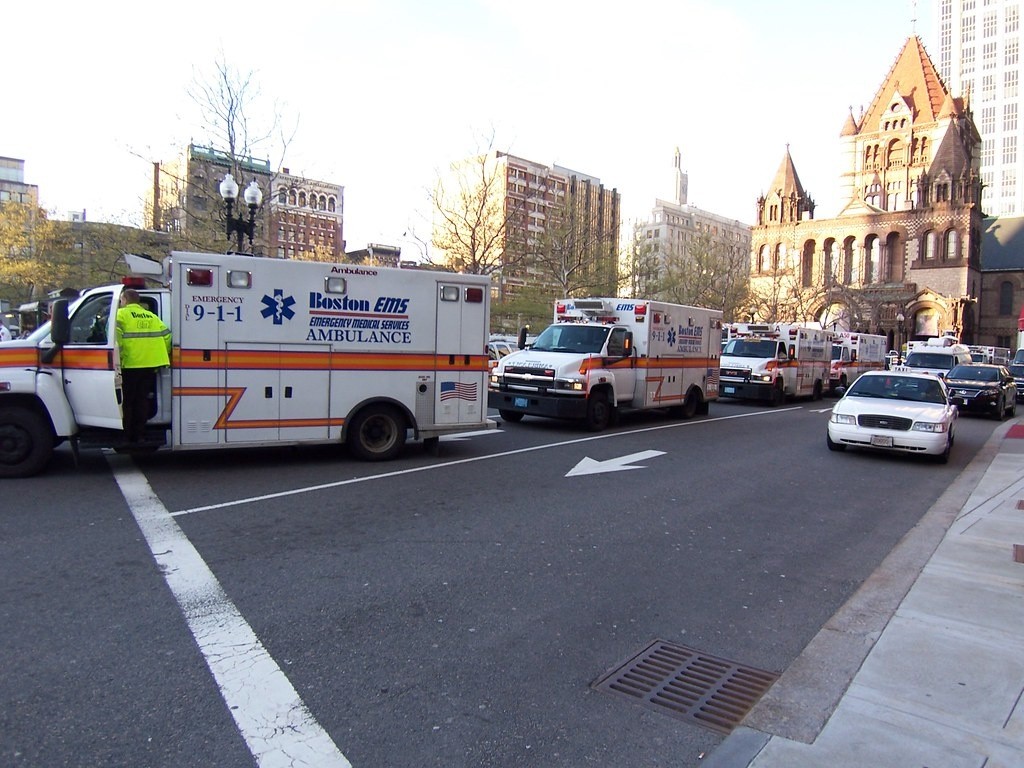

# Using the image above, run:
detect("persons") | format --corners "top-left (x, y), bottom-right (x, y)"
top-left (21, 331), bottom-right (30, 339)
top-left (105, 289), bottom-right (172, 440)
top-left (0, 319), bottom-right (11, 341)
top-left (904, 380), bottom-right (936, 404)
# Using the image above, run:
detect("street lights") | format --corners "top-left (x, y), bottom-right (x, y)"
top-left (750, 307), bottom-right (756, 323)
top-left (219, 173), bottom-right (263, 252)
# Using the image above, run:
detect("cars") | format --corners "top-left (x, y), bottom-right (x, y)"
top-left (900, 335), bottom-right (1024, 421)
top-left (826, 370), bottom-right (959, 464)
top-left (487, 338), bottom-right (518, 361)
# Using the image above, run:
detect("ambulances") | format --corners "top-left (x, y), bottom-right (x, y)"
top-left (0, 250), bottom-right (491, 464)
top-left (718, 324), bottom-right (887, 407)
top-left (487, 297), bottom-right (724, 432)
